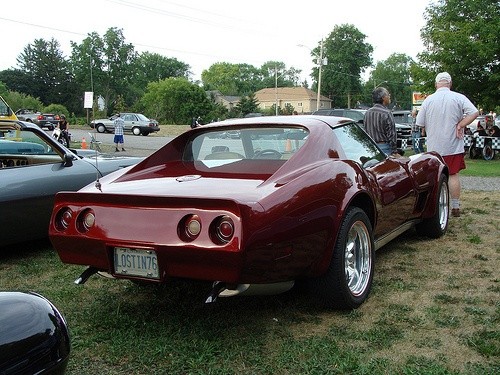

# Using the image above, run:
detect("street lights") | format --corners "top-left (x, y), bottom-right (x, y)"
top-left (295, 44), bottom-right (323, 112)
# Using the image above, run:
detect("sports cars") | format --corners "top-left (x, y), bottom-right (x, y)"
top-left (47, 115), bottom-right (450, 309)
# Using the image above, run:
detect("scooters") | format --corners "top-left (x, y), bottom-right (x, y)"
top-left (50, 123), bottom-right (71, 148)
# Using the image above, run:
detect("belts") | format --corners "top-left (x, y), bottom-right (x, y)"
top-left (377, 141), bottom-right (386, 144)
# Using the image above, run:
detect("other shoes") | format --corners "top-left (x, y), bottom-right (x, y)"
top-left (451, 208), bottom-right (460, 217)
top-left (116, 148), bottom-right (120, 152)
top-left (122, 148), bottom-right (126, 151)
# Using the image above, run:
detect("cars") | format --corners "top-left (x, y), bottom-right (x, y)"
top-left (0, 95), bottom-right (153, 249)
top-left (89, 112), bottom-right (161, 136)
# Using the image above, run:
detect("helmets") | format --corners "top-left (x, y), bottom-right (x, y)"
top-left (60, 114), bottom-right (66, 119)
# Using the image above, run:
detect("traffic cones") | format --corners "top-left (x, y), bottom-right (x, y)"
top-left (81, 134), bottom-right (88, 149)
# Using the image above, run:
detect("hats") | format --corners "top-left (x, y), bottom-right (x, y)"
top-left (435, 72), bottom-right (451, 84)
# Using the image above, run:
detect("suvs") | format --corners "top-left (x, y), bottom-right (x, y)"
top-left (310, 108), bottom-right (500, 156)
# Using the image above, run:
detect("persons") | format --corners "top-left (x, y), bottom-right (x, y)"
top-left (363, 87), bottom-right (401, 157)
top-left (113, 113), bottom-right (127, 152)
top-left (415, 72), bottom-right (479, 217)
top-left (57, 114), bottom-right (70, 148)
top-left (411, 109), bottom-right (425, 153)
top-left (472, 108), bottom-right (500, 136)
top-left (191, 115), bottom-right (204, 129)
